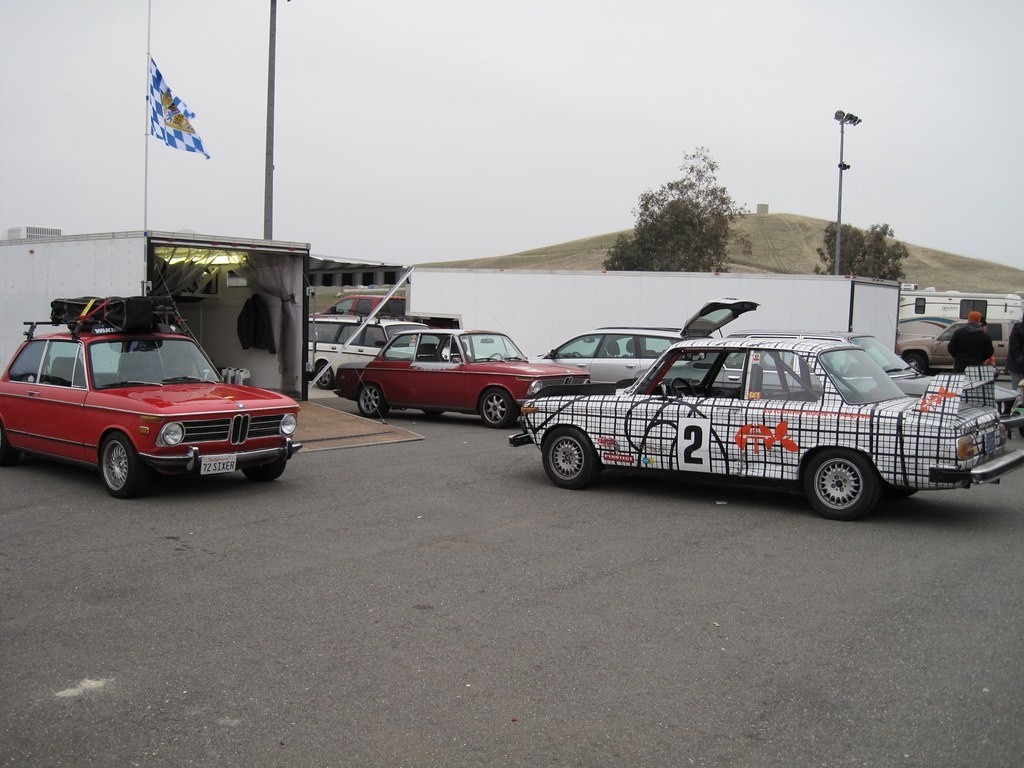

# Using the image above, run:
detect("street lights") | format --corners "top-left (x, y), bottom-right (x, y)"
top-left (834, 111), bottom-right (862, 275)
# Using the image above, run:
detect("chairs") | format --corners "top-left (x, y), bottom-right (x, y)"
top-left (606, 341), bottom-right (620, 357)
top-left (117, 353), bottom-right (158, 381)
top-left (417, 343), bottom-right (440, 361)
top-left (731, 364), bottom-right (763, 398)
top-left (450, 342), bottom-right (473, 361)
top-left (52, 357), bottom-right (85, 386)
top-left (622, 339), bottom-right (635, 357)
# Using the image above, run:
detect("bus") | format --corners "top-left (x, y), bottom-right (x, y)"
top-left (896, 291), bottom-right (1024, 341)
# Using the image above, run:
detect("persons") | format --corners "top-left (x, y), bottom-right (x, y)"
top-left (946, 309), bottom-right (995, 374)
top-left (1006, 312), bottom-right (1024, 388)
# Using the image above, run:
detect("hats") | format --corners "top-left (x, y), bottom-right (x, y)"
top-left (967, 311), bottom-right (982, 323)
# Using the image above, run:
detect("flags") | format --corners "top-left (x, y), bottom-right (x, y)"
top-left (147, 53), bottom-right (210, 161)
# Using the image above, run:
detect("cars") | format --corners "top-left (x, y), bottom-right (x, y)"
top-left (633, 331), bottom-right (1024, 439)
top-left (509, 339), bottom-right (1024, 521)
top-left (332, 328), bottom-right (592, 428)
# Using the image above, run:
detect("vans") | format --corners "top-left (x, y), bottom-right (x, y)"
top-left (308, 295), bottom-right (440, 390)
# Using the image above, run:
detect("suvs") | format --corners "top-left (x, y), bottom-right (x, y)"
top-left (0, 321), bottom-right (302, 500)
top-left (896, 319), bottom-right (1020, 374)
top-left (527, 297), bottom-right (761, 384)
